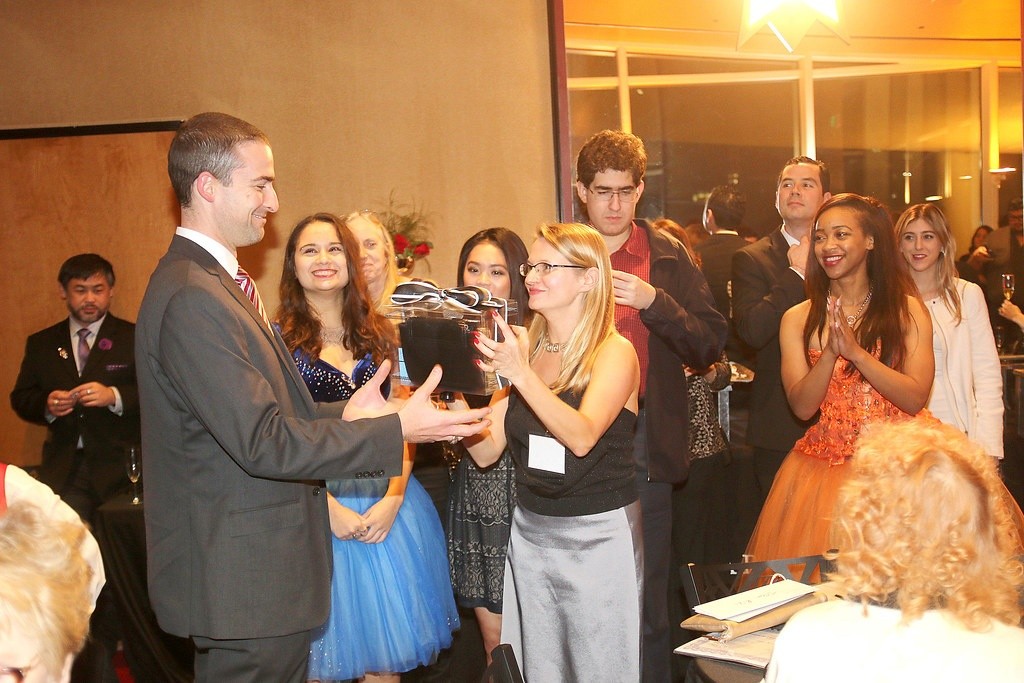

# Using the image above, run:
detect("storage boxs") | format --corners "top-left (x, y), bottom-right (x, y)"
top-left (382, 298), bottom-right (519, 396)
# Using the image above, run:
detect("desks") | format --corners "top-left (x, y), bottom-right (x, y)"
top-left (94, 489), bottom-right (196, 683)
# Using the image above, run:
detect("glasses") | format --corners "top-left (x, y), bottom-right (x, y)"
top-left (519, 262), bottom-right (600, 276)
top-left (582, 181), bottom-right (639, 203)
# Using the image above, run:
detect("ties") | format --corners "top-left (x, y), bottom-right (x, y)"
top-left (235, 265), bottom-right (275, 337)
top-left (77, 328), bottom-right (91, 374)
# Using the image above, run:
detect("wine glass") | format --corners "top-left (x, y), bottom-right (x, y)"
top-left (124, 446), bottom-right (141, 506)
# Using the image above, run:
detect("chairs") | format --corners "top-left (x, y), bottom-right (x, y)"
top-left (679, 549), bottom-right (836, 638)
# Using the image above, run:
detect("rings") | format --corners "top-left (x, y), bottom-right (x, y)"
top-left (54, 400), bottom-right (58, 405)
top-left (87, 389), bottom-right (90, 394)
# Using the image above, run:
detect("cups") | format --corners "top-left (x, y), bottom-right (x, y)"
top-left (1002, 274), bottom-right (1015, 300)
top-left (994, 326), bottom-right (1004, 356)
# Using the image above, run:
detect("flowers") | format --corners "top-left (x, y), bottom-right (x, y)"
top-left (377, 185), bottom-right (434, 276)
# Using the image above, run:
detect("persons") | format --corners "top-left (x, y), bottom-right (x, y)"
top-left (0, 461), bottom-right (107, 683)
top-left (652, 185), bottom-right (764, 312)
top-left (444, 222), bottom-right (641, 683)
top-left (576, 130), bottom-right (729, 683)
top-left (959, 195), bottom-right (1024, 331)
top-left (760, 419), bottom-right (1024, 683)
top-left (894, 204), bottom-right (1004, 466)
top-left (10, 253), bottom-right (136, 523)
top-left (271, 212), bottom-right (460, 683)
top-left (735, 193), bottom-right (942, 588)
top-left (731, 155), bottom-right (832, 507)
top-left (343, 209), bottom-right (437, 323)
top-left (445, 227), bottom-right (530, 683)
top-left (135, 110), bottom-right (492, 683)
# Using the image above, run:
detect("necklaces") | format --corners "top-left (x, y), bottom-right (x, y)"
top-left (541, 332), bottom-right (565, 353)
top-left (927, 295), bottom-right (936, 304)
top-left (846, 287), bottom-right (872, 325)
